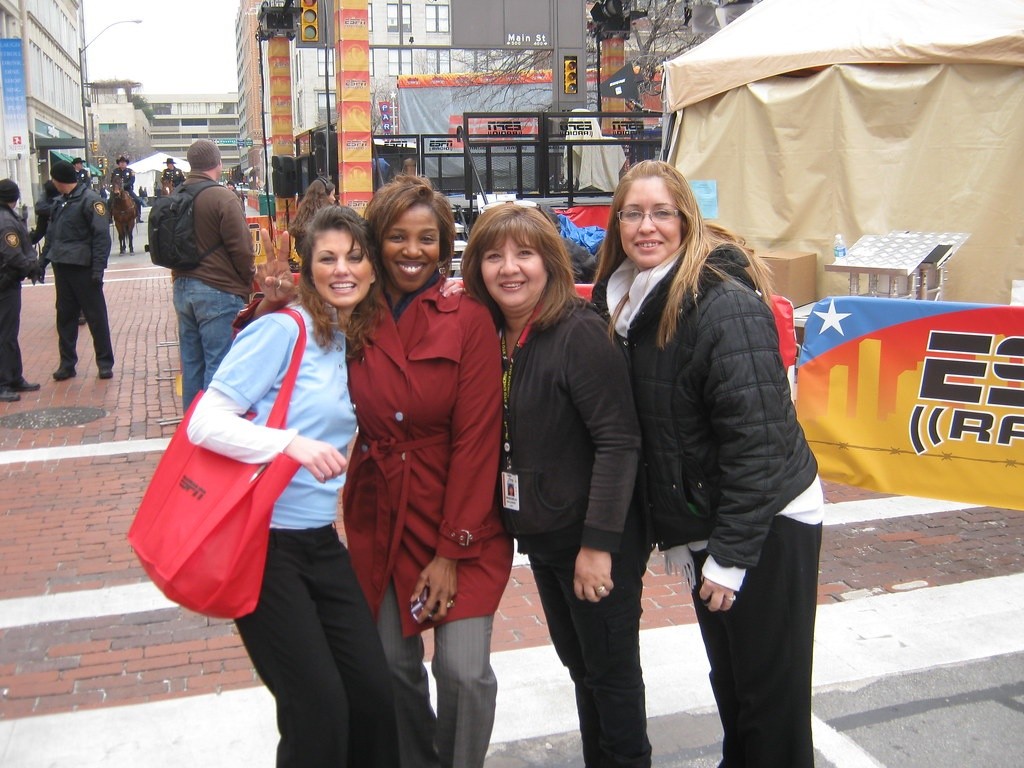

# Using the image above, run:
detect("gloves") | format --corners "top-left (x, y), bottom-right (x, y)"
top-left (28, 229), bottom-right (36, 243)
top-left (27, 262), bottom-right (45, 285)
top-left (91, 271), bottom-right (104, 287)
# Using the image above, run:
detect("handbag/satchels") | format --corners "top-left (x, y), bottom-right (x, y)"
top-left (127, 309), bottom-right (307, 618)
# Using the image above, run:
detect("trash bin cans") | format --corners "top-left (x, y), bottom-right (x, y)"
top-left (259, 194), bottom-right (275, 216)
top-left (148, 197), bottom-right (157, 205)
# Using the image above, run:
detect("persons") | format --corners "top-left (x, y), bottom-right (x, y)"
top-left (228, 181), bottom-right (240, 199)
top-left (72, 158), bottom-right (92, 189)
top-left (592, 160), bottom-right (824, 768)
top-left (462, 203), bottom-right (653, 768)
top-left (289, 177), bottom-right (336, 272)
top-left (161, 158), bottom-right (185, 190)
top-left (0, 179), bottom-right (39, 401)
top-left (171, 140), bottom-right (256, 414)
top-left (100, 186), bottom-right (106, 201)
top-left (107, 157), bottom-right (144, 223)
top-left (37, 161), bottom-right (114, 379)
top-left (35, 180), bottom-right (87, 325)
top-left (232, 176), bottom-right (513, 768)
top-left (508, 484), bottom-right (514, 496)
top-left (139, 186), bottom-right (148, 204)
top-left (187, 206), bottom-right (465, 768)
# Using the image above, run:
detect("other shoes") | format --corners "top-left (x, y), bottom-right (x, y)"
top-left (52, 366), bottom-right (76, 380)
top-left (8, 376), bottom-right (40, 392)
top-left (109, 220), bottom-right (113, 224)
top-left (99, 368), bottom-right (114, 378)
top-left (0, 391), bottom-right (20, 402)
top-left (79, 316), bottom-right (86, 325)
top-left (136, 219), bottom-right (143, 223)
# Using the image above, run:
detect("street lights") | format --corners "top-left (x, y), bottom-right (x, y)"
top-left (78, 20), bottom-right (143, 191)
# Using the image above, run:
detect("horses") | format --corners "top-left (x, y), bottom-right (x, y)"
top-left (160, 173), bottom-right (174, 197)
top-left (108, 172), bottom-right (139, 257)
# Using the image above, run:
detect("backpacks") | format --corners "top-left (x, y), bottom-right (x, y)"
top-left (148, 181), bottom-right (227, 271)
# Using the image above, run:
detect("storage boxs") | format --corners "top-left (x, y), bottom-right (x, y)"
top-left (746, 250), bottom-right (817, 308)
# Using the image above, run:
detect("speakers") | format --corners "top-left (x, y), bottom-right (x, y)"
top-left (272, 155), bottom-right (297, 199)
top-left (314, 130), bottom-right (340, 197)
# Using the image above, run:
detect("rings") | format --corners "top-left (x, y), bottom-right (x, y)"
top-left (276, 280), bottom-right (282, 289)
top-left (596, 585), bottom-right (605, 592)
top-left (447, 600), bottom-right (454, 607)
top-left (726, 595), bottom-right (736, 600)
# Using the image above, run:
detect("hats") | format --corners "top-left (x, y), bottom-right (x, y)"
top-left (71, 158), bottom-right (86, 164)
top-left (0, 178), bottom-right (20, 203)
top-left (163, 158), bottom-right (177, 164)
top-left (50, 160), bottom-right (77, 184)
top-left (187, 140), bottom-right (220, 171)
top-left (116, 156), bottom-right (129, 165)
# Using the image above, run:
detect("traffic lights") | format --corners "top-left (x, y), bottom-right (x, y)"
top-left (98, 157), bottom-right (103, 169)
top-left (294, 0), bottom-right (325, 48)
top-left (92, 141), bottom-right (98, 152)
top-left (104, 158), bottom-right (108, 168)
top-left (564, 55), bottom-right (578, 94)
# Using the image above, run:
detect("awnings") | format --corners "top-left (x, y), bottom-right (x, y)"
top-left (51, 151), bottom-right (102, 175)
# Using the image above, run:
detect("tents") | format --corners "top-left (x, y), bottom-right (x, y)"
top-left (661, 0), bottom-right (1024, 304)
top-left (127, 153), bottom-right (191, 197)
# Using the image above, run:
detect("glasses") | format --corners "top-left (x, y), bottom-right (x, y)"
top-left (617, 206), bottom-right (680, 225)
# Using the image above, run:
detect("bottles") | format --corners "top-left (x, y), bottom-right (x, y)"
top-left (834, 233), bottom-right (846, 264)
